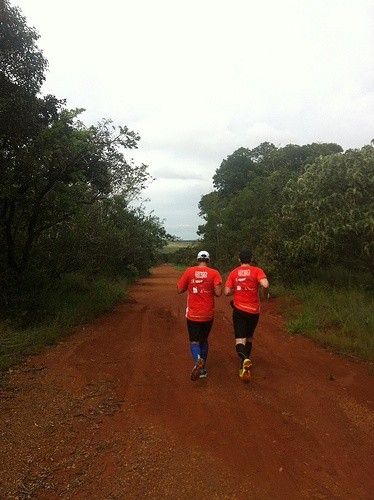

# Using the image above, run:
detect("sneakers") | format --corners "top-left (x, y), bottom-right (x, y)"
top-left (199, 369), bottom-right (208, 378)
top-left (191, 358), bottom-right (204, 381)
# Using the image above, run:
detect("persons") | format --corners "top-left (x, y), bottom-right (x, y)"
top-left (224, 249), bottom-right (270, 384)
top-left (177, 250), bottom-right (223, 381)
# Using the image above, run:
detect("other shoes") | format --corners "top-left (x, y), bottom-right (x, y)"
top-left (239, 368), bottom-right (243, 377)
top-left (242, 359), bottom-right (252, 383)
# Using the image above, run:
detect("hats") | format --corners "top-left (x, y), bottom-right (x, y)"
top-left (197, 251), bottom-right (211, 263)
top-left (239, 249), bottom-right (252, 260)
